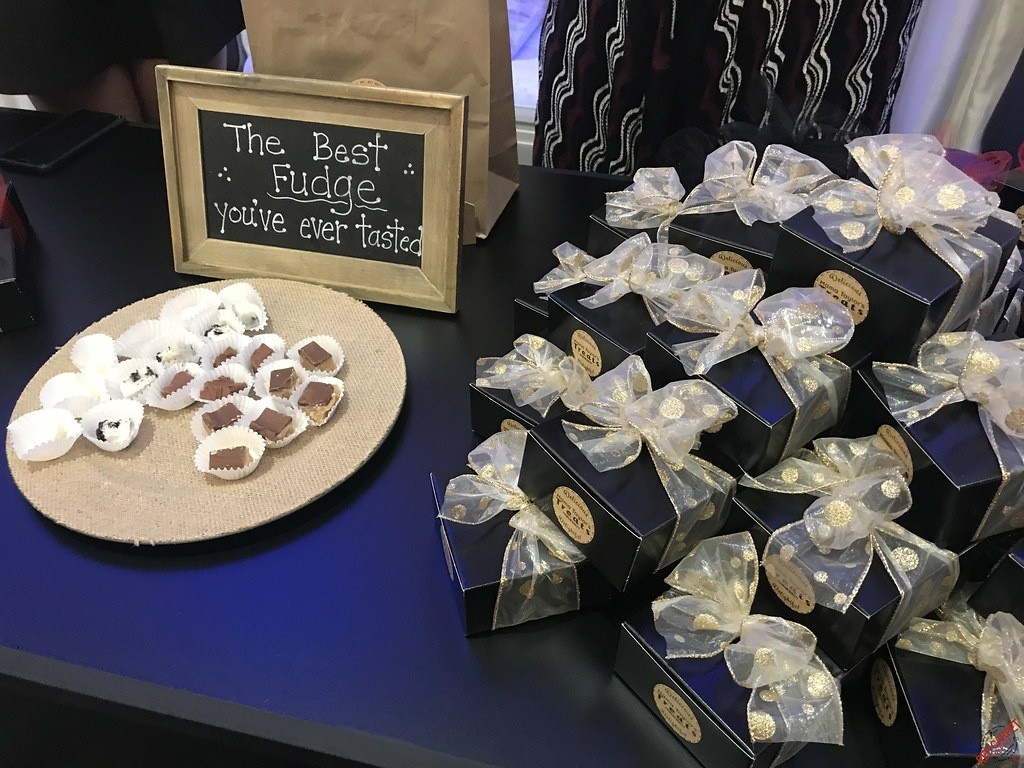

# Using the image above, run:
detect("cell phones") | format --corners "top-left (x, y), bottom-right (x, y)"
top-left (0, 108), bottom-right (126, 176)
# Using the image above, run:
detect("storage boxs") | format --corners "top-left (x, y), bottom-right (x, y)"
top-left (430, 428), bottom-right (594, 638)
top-left (870, 600), bottom-right (1024, 768)
top-left (514, 241), bottom-right (600, 337)
top-left (588, 167), bottom-right (687, 261)
top-left (668, 140), bottom-right (842, 278)
top-left (643, 267), bottom-right (879, 499)
top-left (0, 229), bottom-right (36, 336)
top-left (468, 333), bottom-right (591, 439)
top-left (552, 234), bottom-right (725, 382)
top-left (613, 530), bottom-right (855, 767)
top-left (965, 537), bottom-right (1024, 631)
top-left (0, 174), bottom-right (34, 257)
top-left (517, 353), bottom-right (746, 594)
top-left (725, 434), bottom-right (983, 672)
top-left (768, 132), bottom-right (1020, 366)
top-left (857, 329), bottom-right (1024, 556)
top-left (944, 147), bottom-right (1024, 343)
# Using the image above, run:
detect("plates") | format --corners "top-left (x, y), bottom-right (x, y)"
top-left (4, 277), bottom-right (407, 544)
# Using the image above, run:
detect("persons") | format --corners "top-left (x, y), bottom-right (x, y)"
top-left (1, 0), bottom-right (248, 124)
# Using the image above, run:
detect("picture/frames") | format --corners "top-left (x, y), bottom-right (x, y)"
top-left (153, 61), bottom-right (471, 318)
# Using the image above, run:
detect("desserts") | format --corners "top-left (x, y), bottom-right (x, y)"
top-left (12, 280), bottom-right (344, 478)
